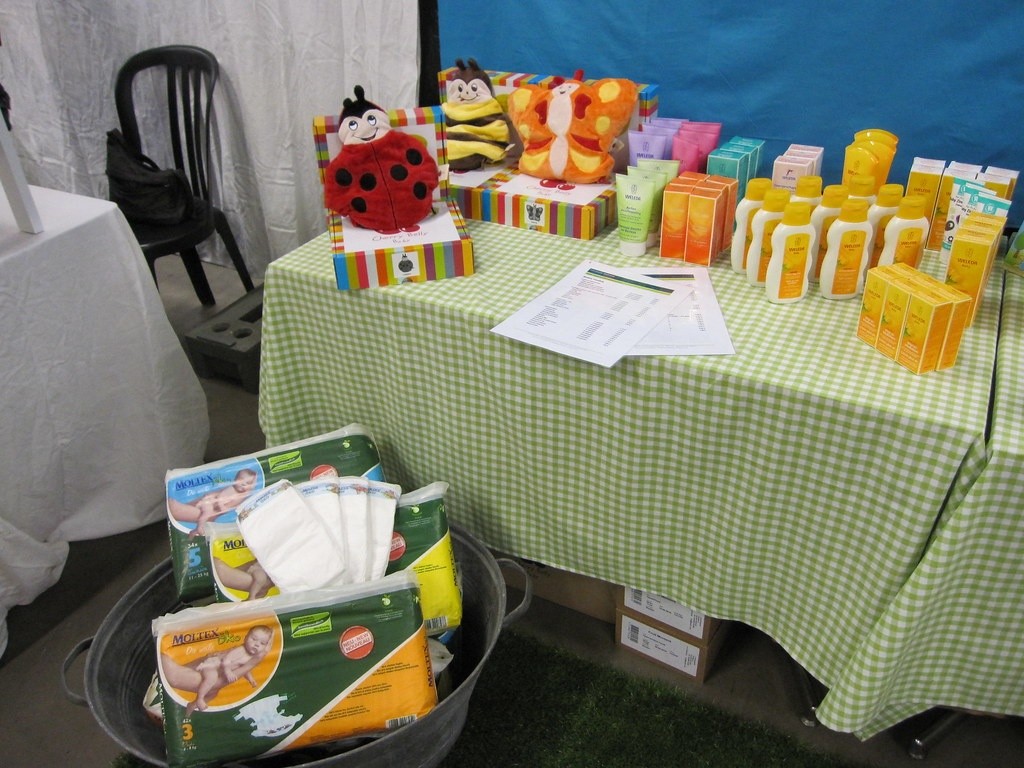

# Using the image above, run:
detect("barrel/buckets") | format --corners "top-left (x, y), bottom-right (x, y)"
top-left (60, 519), bottom-right (532, 768)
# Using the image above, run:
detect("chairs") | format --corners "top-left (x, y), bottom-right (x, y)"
top-left (116, 44), bottom-right (256, 310)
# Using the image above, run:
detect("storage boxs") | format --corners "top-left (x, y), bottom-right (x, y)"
top-left (489, 548), bottom-right (733, 685)
top-left (313, 64), bottom-right (1021, 375)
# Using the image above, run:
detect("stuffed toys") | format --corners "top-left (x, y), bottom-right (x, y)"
top-left (440, 57), bottom-right (510, 170)
top-left (323, 85), bottom-right (438, 231)
top-left (508, 68), bottom-right (639, 184)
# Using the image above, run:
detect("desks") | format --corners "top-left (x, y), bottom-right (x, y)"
top-left (907, 232), bottom-right (1024, 759)
top-left (0, 179), bottom-right (211, 657)
top-left (256, 215), bottom-right (1009, 744)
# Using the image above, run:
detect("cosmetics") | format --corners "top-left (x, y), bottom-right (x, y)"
top-left (612, 117), bottom-right (1020, 376)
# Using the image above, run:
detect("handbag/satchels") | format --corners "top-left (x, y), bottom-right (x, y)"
top-left (104, 128), bottom-right (204, 228)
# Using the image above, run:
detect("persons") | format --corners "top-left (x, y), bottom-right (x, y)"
top-left (162, 625), bottom-right (274, 717)
top-left (212, 556), bottom-right (275, 601)
top-left (168, 468), bottom-right (258, 540)
